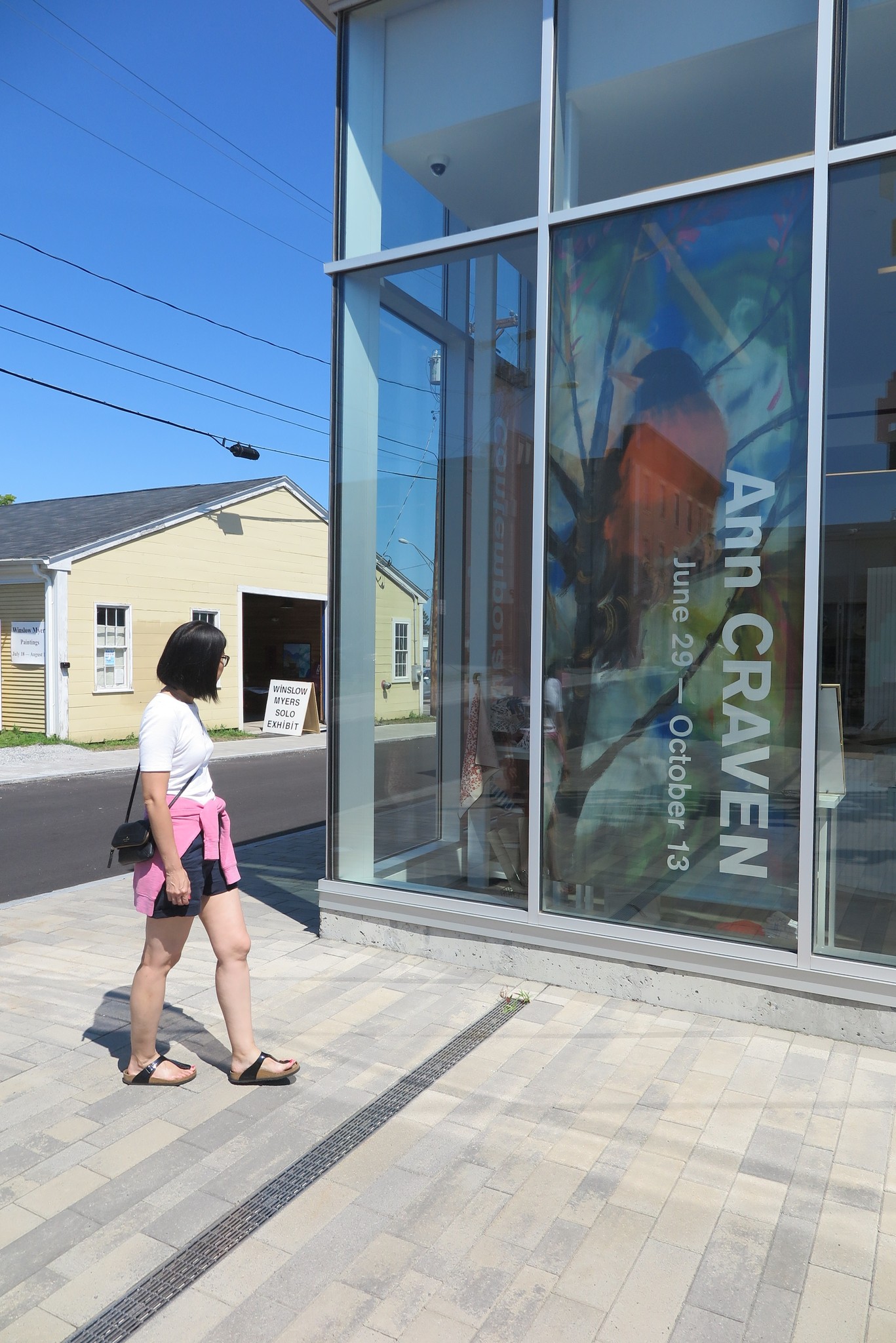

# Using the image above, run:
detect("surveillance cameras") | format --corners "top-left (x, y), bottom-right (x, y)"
top-left (428, 154), bottom-right (450, 177)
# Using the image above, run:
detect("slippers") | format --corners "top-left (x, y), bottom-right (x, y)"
top-left (121, 1055), bottom-right (197, 1085)
top-left (229, 1051), bottom-right (300, 1083)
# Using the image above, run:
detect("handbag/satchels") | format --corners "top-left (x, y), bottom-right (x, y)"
top-left (106, 818), bottom-right (154, 868)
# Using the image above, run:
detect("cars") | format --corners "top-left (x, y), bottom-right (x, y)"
top-left (423, 667), bottom-right (431, 700)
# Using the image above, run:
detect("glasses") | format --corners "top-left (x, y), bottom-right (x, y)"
top-left (221, 654), bottom-right (230, 667)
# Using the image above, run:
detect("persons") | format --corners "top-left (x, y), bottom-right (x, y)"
top-left (123, 620), bottom-right (301, 1086)
top-left (542, 658), bottom-right (565, 848)
